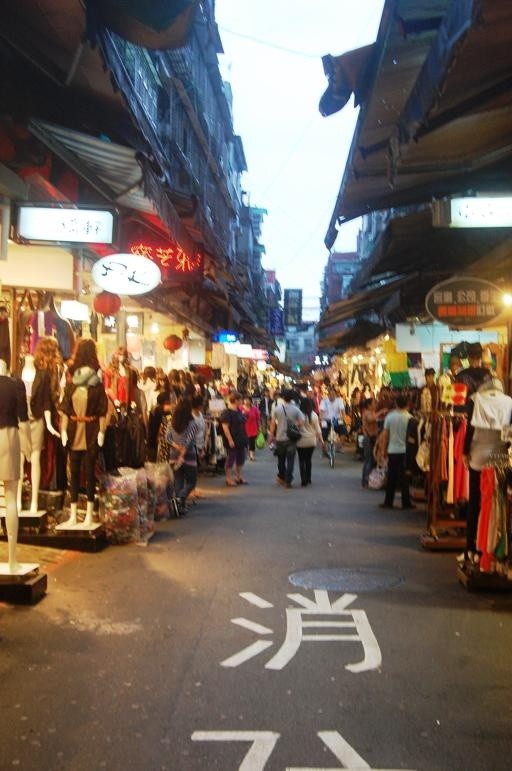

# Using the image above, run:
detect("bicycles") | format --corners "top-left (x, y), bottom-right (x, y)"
top-left (319, 415), bottom-right (345, 466)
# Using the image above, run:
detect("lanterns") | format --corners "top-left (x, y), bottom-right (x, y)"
top-left (94, 290), bottom-right (122, 318)
top-left (163, 335), bottom-right (183, 354)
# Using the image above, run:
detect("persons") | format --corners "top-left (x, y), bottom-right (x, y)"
top-left (0, 336), bottom-right (157, 568)
top-left (436, 343), bottom-right (511, 562)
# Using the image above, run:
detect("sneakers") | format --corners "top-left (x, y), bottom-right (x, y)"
top-left (171, 497), bottom-right (185, 516)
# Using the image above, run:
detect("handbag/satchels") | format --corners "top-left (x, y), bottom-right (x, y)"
top-left (282, 407), bottom-right (301, 441)
top-left (368, 456), bottom-right (388, 489)
top-left (256, 429), bottom-right (267, 449)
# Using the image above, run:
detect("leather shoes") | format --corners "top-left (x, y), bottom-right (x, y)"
top-left (225, 478), bottom-right (237, 487)
top-left (378, 503), bottom-right (393, 508)
top-left (236, 477), bottom-right (248, 485)
top-left (403, 503), bottom-right (416, 508)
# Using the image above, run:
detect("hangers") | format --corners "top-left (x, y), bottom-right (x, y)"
top-left (484, 454), bottom-right (511, 476)
top-left (411, 410), bottom-right (467, 422)
